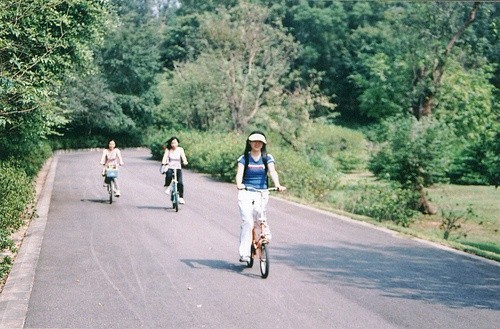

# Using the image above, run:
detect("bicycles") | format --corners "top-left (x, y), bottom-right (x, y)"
top-left (163, 163), bottom-right (186, 211)
top-left (240, 185), bottom-right (282, 276)
top-left (101, 163), bottom-right (121, 204)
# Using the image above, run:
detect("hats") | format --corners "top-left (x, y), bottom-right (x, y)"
top-left (248, 133), bottom-right (267, 144)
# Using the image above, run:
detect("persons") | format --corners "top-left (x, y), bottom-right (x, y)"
top-left (100, 138), bottom-right (123, 197)
top-left (236, 130), bottom-right (284, 261)
top-left (162, 136), bottom-right (188, 204)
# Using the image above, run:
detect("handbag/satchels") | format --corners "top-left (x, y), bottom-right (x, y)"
top-left (102, 165), bottom-right (107, 176)
top-left (160, 157), bottom-right (169, 174)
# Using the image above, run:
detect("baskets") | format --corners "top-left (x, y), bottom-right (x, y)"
top-left (106, 168), bottom-right (118, 177)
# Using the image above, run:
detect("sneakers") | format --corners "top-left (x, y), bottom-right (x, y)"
top-left (241, 256), bottom-right (251, 261)
top-left (164, 186), bottom-right (170, 195)
top-left (115, 191), bottom-right (120, 196)
top-left (179, 197), bottom-right (184, 204)
top-left (103, 182), bottom-right (109, 187)
top-left (265, 232), bottom-right (272, 240)
